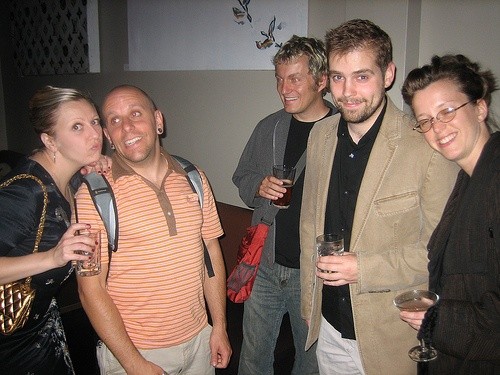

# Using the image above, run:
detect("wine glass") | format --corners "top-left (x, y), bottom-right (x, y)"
top-left (393, 289), bottom-right (440, 362)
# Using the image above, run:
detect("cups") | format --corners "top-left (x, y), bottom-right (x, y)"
top-left (316, 234), bottom-right (344, 282)
top-left (271, 165), bottom-right (296, 209)
top-left (74, 228), bottom-right (101, 277)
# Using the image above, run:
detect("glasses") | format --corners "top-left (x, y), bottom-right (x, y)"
top-left (412, 99), bottom-right (474, 133)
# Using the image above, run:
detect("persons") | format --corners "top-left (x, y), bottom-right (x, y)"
top-left (399, 53), bottom-right (500, 375)
top-left (231, 34), bottom-right (338, 375)
top-left (71, 84), bottom-right (233, 375)
top-left (0, 85), bottom-right (103, 375)
top-left (299, 18), bottom-right (462, 375)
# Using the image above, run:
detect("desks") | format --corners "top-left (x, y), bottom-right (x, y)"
top-left (55, 288), bottom-right (83, 313)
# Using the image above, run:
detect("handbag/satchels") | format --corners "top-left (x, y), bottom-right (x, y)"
top-left (226, 223), bottom-right (270, 304)
top-left (0, 173), bottom-right (49, 336)
